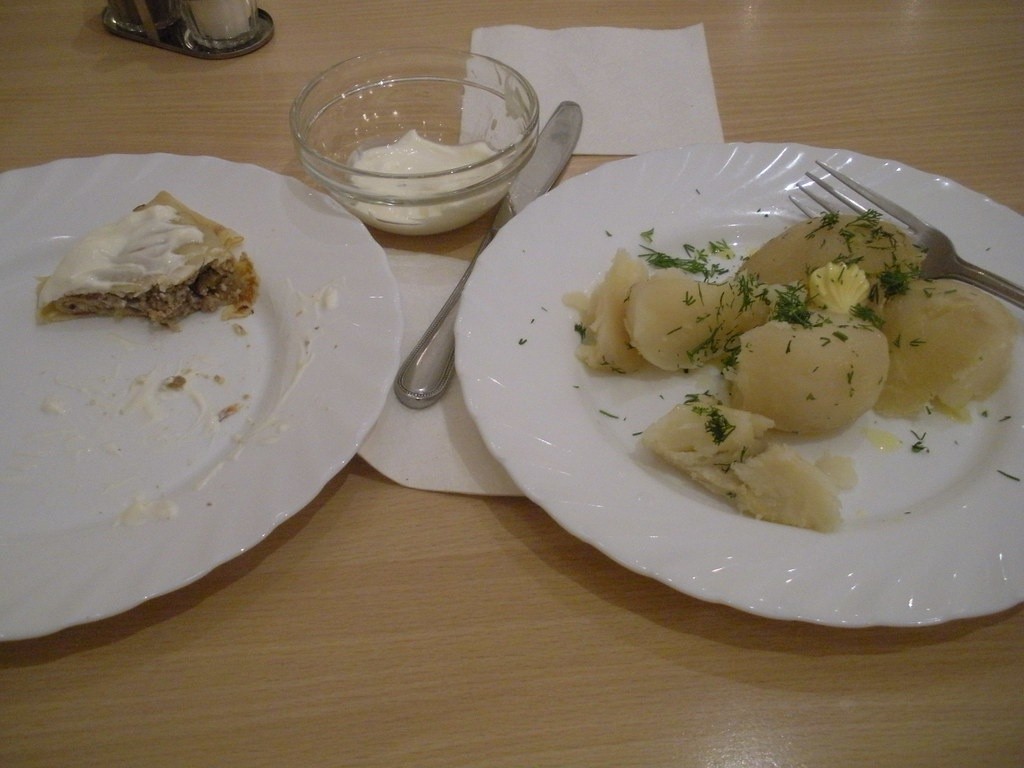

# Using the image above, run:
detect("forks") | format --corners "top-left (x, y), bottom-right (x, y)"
top-left (788, 159), bottom-right (1024, 312)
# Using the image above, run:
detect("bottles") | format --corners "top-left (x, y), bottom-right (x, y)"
top-left (106, 0), bottom-right (178, 30)
top-left (176, 0), bottom-right (257, 49)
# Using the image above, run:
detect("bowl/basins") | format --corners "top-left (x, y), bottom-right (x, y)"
top-left (289, 46), bottom-right (538, 235)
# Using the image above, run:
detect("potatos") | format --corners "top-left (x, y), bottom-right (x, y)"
top-left (563, 212), bottom-right (1020, 534)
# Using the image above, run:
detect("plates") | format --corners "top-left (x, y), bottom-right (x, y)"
top-left (453, 142), bottom-right (1024, 629)
top-left (0, 152), bottom-right (403, 642)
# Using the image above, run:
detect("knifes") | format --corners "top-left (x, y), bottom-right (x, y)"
top-left (395, 100), bottom-right (583, 410)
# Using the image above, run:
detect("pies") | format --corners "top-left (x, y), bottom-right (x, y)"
top-left (31, 190), bottom-right (261, 332)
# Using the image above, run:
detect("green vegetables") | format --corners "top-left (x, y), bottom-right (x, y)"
top-left (518, 187), bottom-right (966, 451)
